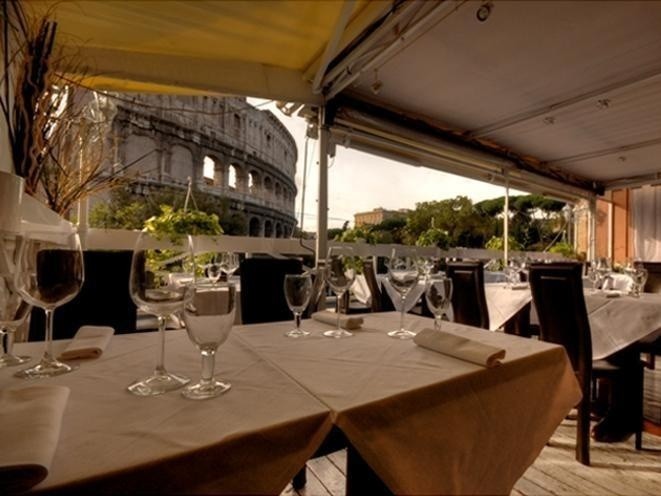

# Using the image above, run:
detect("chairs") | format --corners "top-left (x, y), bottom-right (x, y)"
top-left (634, 260), bottom-right (660, 372)
top-left (25, 245), bottom-right (137, 344)
top-left (239, 256), bottom-right (309, 489)
top-left (527, 261), bottom-right (653, 466)
top-left (361, 259), bottom-right (397, 313)
top-left (444, 262), bottom-right (491, 330)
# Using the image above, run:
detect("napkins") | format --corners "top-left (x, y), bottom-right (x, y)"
top-left (0, 386), bottom-right (70, 484)
top-left (413, 327), bottom-right (507, 369)
top-left (58, 325), bottom-right (115, 362)
top-left (512, 285), bottom-right (528, 290)
top-left (311, 308), bottom-right (365, 330)
top-left (605, 293), bottom-right (621, 299)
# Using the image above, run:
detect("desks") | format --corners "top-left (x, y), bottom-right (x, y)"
top-left (480, 281), bottom-right (661, 427)
top-left (166, 272), bottom-right (355, 327)
top-left (350, 269), bottom-right (513, 323)
top-left (0, 310), bottom-right (584, 495)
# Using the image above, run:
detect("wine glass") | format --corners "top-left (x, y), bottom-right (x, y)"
top-left (387, 248), bottom-right (421, 340)
top-left (181, 281), bottom-right (239, 402)
top-left (324, 247), bottom-right (357, 339)
top-left (15, 229), bottom-right (85, 380)
top-left (0, 232), bottom-right (34, 369)
top-left (208, 252), bottom-right (241, 288)
top-left (426, 277), bottom-right (453, 331)
top-left (484, 257), bottom-right (650, 297)
top-left (124, 230), bottom-right (195, 398)
top-left (284, 274), bottom-right (312, 339)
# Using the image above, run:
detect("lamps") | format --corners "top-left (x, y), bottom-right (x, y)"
top-left (475, 0), bottom-right (495, 22)
top-left (369, 68), bottom-right (383, 94)
